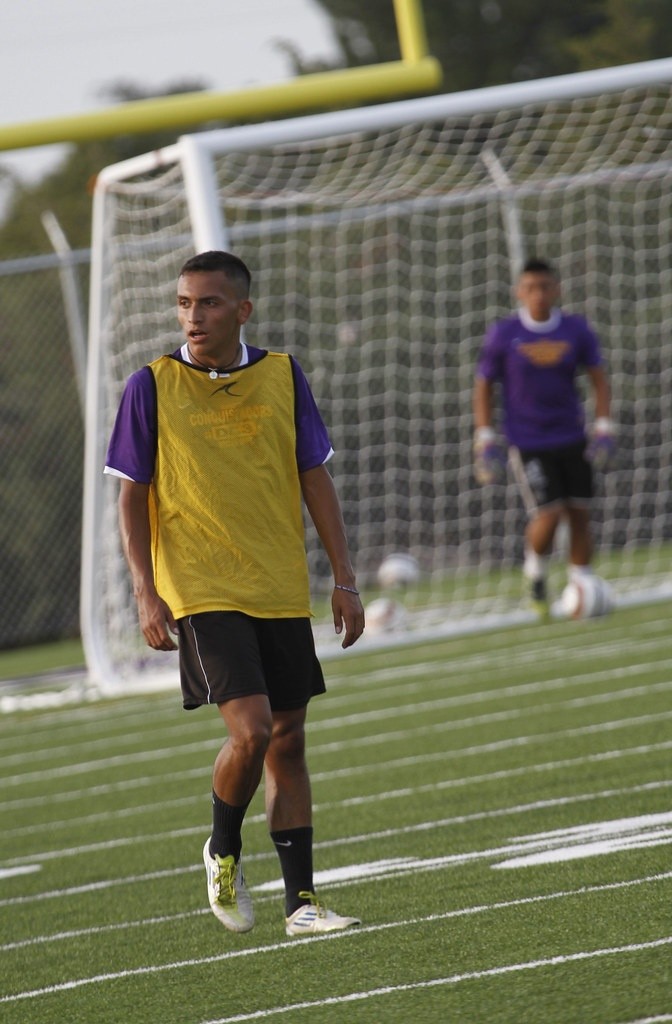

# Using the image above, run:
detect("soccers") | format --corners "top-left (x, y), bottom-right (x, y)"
top-left (560, 574), bottom-right (615, 620)
top-left (364, 598), bottom-right (407, 645)
top-left (377, 552), bottom-right (421, 590)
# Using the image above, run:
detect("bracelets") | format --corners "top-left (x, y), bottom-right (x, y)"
top-left (594, 416), bottom-right (611, 432)
top-left (473, 426), bottom-right (494, 442)
top-left (335, 585), bottom-right (360, 595)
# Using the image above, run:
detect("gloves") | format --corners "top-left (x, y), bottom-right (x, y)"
top-left (474, 425), bottom-right (509, 485)
top-left (584, 416), bottom-right (618, 475)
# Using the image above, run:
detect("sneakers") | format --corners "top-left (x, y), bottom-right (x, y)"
top-left (284, 904), bottom-right (362, 936)
top-left (523, 565), bottom-right (549, 616)
top-left (203, 834), bottom-right (256, 933)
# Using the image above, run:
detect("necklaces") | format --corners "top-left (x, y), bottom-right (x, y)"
top-left (187, 347), bottom-right (240, 379)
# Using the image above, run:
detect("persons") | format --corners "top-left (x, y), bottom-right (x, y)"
top-left (103, 251), bottom-right (366, 936)
top-left (472, 258), bottom-right (618, 620)
